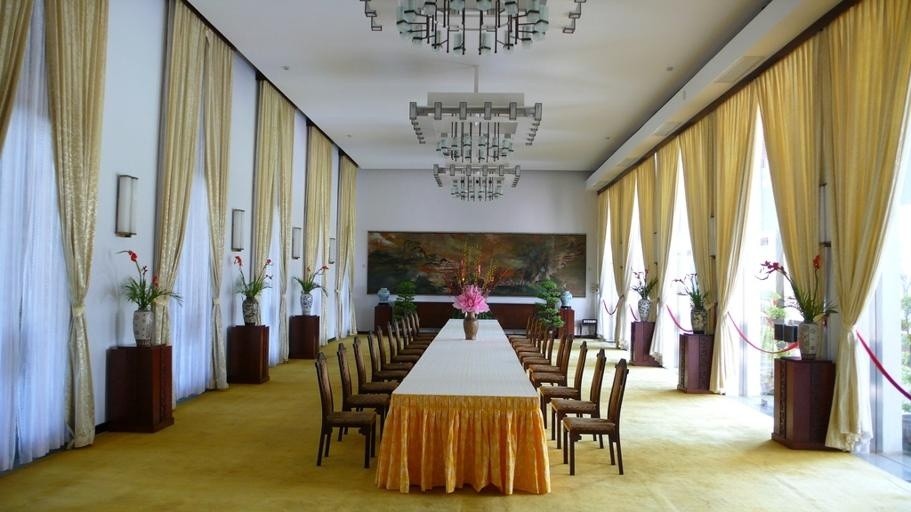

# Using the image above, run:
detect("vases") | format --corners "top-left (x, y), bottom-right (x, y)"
top-left (462, 311), bottom-right (480, 340)
top-left (132, 310), bottom-right (154, 346)
top-left (689, 308), bottom-right (707, 334)
top-left (638, 299), bottom-right (651, 321)
top-left (300, 294), bottom-right (313, 315)
top-left (378, 287), bottom-right (393, 304)
top-left (243, 299), bottom-right (260, 327)
top-left (562, 291), bottom-right (574, 307)
top-left (799, 322), bottom-right (819, 360)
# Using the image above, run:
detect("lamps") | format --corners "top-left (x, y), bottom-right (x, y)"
top-left (292, 227), bottom-right (302, 259)
top-left (361, 1), bottom-right (587, 57)
top-left (114, 174), bottom-right (140, 237)
top-left (433, 164), bottom-right (521, 203)
top-left (329, 237), bottom-right (337, 264)
top-left (232, 208), bottom-right (245, 251)
top-left (407, 65), bottom-right (543, 163)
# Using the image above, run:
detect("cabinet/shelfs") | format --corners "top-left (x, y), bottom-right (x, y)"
top-left (225, 325), bottom-right (271, 385)
top-left (675, 333), bottom-right (721, 393)
top-left (628, 321), bottom-right (662, 367)
top-left (289, 315), bottom-right (320, 359)
top-left (374, 302), bottom-right (393, 335)
top-left (771, 357), bottom-right (841, 450)
top-left (557, 308), bottom-right (575, 338)
top-left (105, 343), bottom-right (174, 434)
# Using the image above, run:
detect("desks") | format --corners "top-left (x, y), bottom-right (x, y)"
top-left (374, 319), bottom-right (552, 495)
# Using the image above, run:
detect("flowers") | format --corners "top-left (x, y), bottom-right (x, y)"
top-left (631, 269), bottom-right (658, 299)
top-left (754, 241), bottom-right (839, 323)
top-left (230, 256), bottom-right (273, 299)
top-left (114, 248), bottom-right (185, 310)
top-left (673, 273), bottom-right (709, 309)
top-left (291, 264), bottom-right (329, 296)
top-left (448, 283), bottom-right (490, 313)
